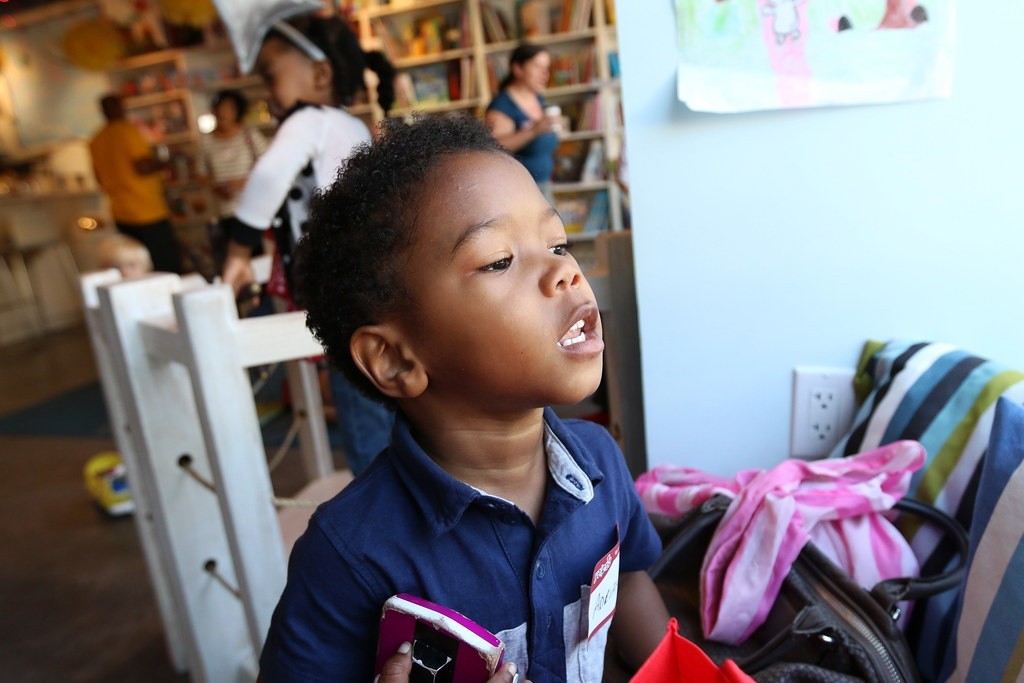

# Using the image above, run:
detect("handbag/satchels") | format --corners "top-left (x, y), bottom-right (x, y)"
top-left (649, 493), bottom-right (972, 683)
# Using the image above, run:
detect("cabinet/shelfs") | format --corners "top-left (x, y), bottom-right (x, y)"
top-left (102, 0), bottom-right (632, 284)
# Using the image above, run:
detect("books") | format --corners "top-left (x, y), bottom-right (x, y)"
top-left (374, 0), bottom-right (614, 235)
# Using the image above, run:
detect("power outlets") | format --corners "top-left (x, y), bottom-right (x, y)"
top-left (791, 366), bottom-right (857, 458)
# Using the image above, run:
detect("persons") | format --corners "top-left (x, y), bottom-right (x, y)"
top-left (92, 94), bottom-right (187, 273)
top-left (219, 14), bottom-right (395, 477)
top-left (255, 116), bottom-right (672, 683)
top-left (487, 45), bottom-right (569, 204)
top-left (196, 88), bottom-right (269, 298)
